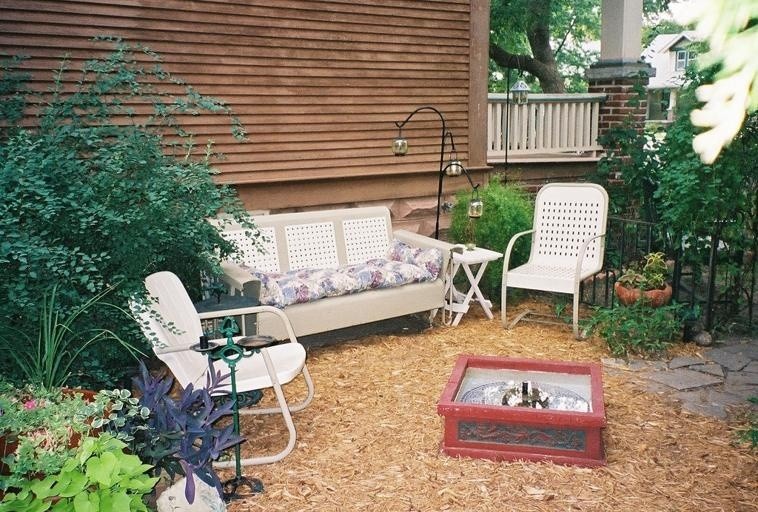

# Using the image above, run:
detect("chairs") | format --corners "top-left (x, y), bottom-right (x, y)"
top-left (133, 272), bottom-right (314, 466)
top-left (500, 181), bottom-right (608, 341)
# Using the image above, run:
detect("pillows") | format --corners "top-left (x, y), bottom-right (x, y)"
top-left (246, 240), bottom-right (442, 306)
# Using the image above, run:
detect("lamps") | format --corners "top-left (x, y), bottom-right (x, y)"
top-left (393, 107), bottom-right (484, 240)
top-left (505, 55), bottom-right (531, 184)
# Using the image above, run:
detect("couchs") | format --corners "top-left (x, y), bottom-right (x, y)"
top-left (198, 204), bottom-right (449, 342)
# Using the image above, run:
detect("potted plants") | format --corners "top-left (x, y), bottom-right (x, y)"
top-left (615, 252), bottom-right (673, 306)
top-left (0, 378), bottom-right (158, 495)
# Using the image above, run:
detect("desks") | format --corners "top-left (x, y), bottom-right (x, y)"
top-left (446, 244), bottom-right (502, 325)
